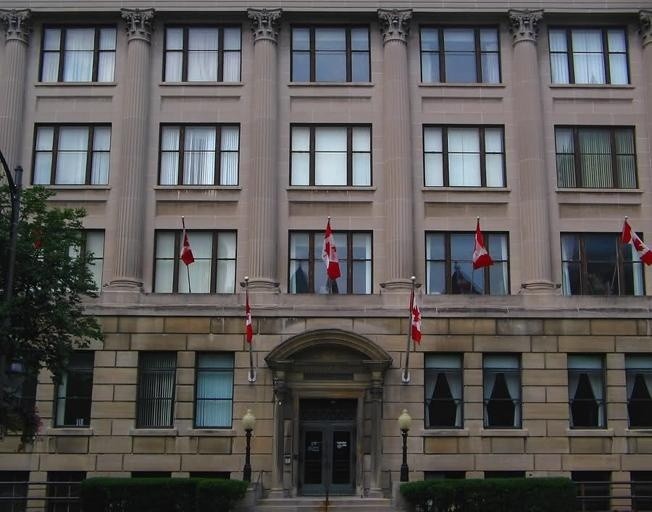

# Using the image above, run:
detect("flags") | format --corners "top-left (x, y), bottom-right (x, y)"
top-left (178, 226), bottom-right (195, 266)
top-left (321, 219), bottom-right (341, 280)
top-left (472, 222), bottom-right (495, 269)
top-left (617, 220), bottom-right (652, 267)
top-left (408, 288), bottom-right (424, 345)
top-left (244, 289), bottom-right (253, 343)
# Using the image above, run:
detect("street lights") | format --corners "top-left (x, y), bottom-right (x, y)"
top-left (398, 409), bottom-right (412, 482)
top-left (242, 408), bottom-right (256, 482)
